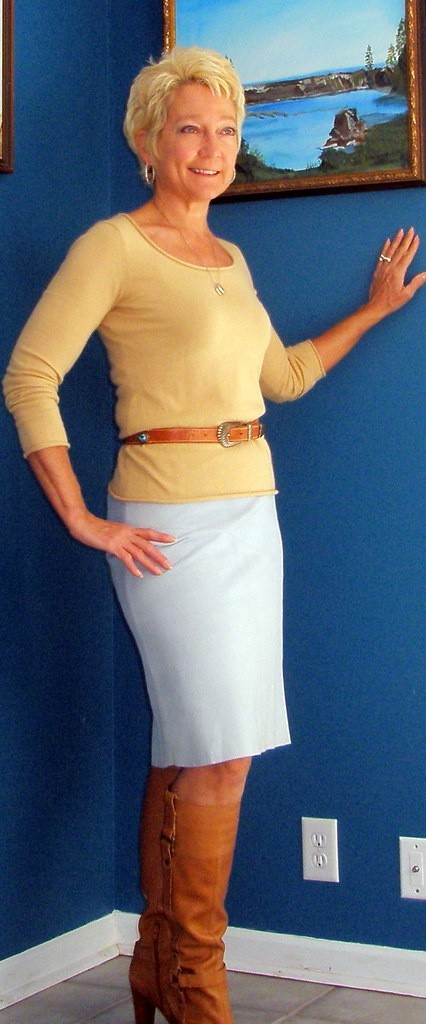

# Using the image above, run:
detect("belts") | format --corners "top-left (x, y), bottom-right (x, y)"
top-left (125, 418), bottom-right (262, 448)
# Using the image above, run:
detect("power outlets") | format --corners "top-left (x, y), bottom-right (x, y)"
top-left (301, 817), bottom-right (339, 883)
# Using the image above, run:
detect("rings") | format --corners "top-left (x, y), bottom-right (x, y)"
top-left (378, 254), bottom-right (384, 262)
top-left (383, 257), bottom-right (391, 262)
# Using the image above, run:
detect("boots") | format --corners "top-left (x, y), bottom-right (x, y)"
top-left (128, 765), bottom-right (173, 1024)
top-left (158, 790), bottom-right (241, 1024)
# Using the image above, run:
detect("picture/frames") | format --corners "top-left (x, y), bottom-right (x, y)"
top-left (0, 0), bottom-right (14, 175)
top-left (163, 0), bottom-right (426, 199)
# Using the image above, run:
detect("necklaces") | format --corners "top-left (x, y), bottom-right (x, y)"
top-left (152, 201), bottom-right (224, 296)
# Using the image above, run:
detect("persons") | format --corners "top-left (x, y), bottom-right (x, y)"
top-left (0, 46), bottom-right (426, 1024)
